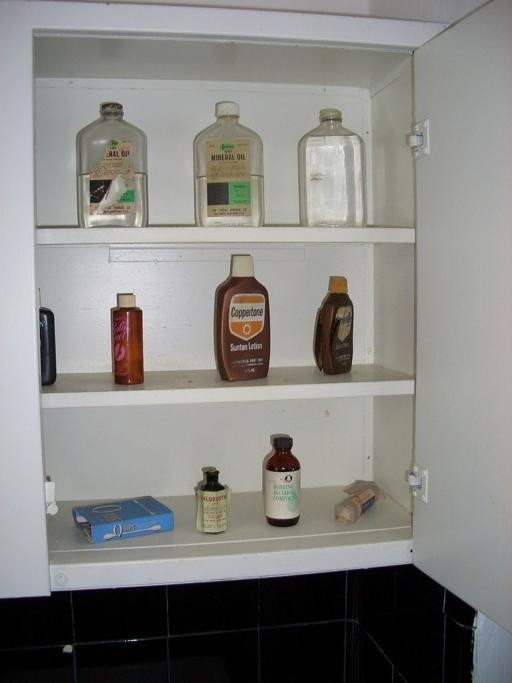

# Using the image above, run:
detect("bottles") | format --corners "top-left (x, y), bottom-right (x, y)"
top-left (335, 486), bottom-right (376, 523)
top-left (193, 99), bottom-right (265, 228)
top-left (110, 292), bottom-right (146, 385)
top-left (296, 108), bottom-right (368, 226)
top-left (75, 101), bottom-right (150, 228)
top-left (315, 276), bottom-right (354, 373)
top-left (215, 253), bottom-right (270, 380)
top-left (37, 288), bottom-right (57, 386)
top-left (264, 438), bottom-right (302, 527)
top-left (195, 469), bottom-right (229, 533)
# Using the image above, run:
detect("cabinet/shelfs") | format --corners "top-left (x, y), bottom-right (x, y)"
top-left (1, 0), bottom-right (512, 636)
